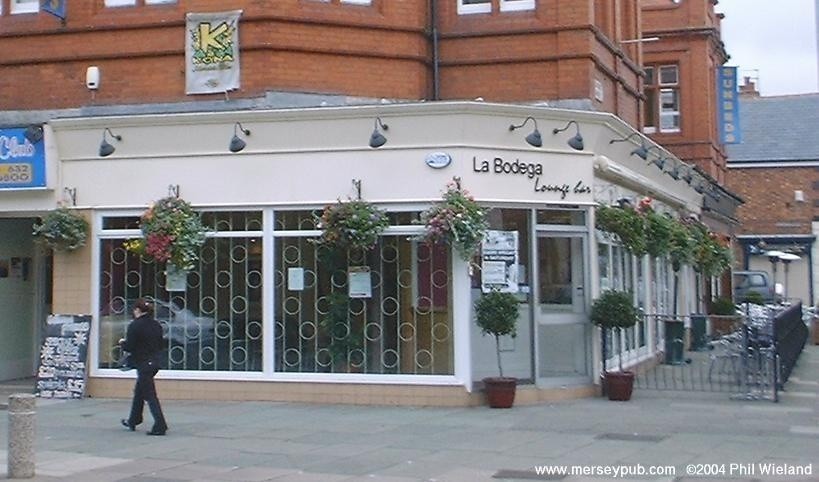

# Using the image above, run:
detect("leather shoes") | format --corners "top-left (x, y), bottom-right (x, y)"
top-left (146, 430), bottom-right (165, 435)
top-left (122, 419), bottom-right (135, 431)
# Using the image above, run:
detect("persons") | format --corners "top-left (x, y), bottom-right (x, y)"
top-left (118, 297), bottom-right (168, 436)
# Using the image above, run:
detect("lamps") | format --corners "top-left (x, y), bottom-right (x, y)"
top-left (99, 128), bottom-right (121, 156)
top-left (610, 133), bottom-right (720, 202)
top-left (509, 116), bottom-right (583, 150)
top-left (369, 117), bottom-right (388, 147)
top-left (230, 122), bottom-right (250, 152)
top-left (24, 122), bottom-right (45, 143)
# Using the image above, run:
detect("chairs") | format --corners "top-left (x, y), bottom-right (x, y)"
top-left (707, 303), bottom-right (785, 386)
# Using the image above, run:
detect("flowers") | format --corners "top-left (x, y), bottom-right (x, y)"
top-left (635, 195), bottom-right (730, 278)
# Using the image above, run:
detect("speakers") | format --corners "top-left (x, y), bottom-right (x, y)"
top-left (87, 66), bottom-right (100, 89)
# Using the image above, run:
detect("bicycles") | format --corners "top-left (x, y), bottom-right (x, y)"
top-left (707, 312), bottom-right (781, 388)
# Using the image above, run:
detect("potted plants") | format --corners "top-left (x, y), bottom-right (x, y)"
top-left (474, 290), bottom-right (520, 407)
top-left (589, 287), bottom-right (639, 401)
top-left (708, 297), bottom-right (735, 338)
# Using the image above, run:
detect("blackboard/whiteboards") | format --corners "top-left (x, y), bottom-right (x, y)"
top-left (32, 314), bottom-right (92, 399)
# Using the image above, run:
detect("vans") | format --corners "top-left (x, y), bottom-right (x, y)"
top-left (733, 269), bottom-right (776, 306)
